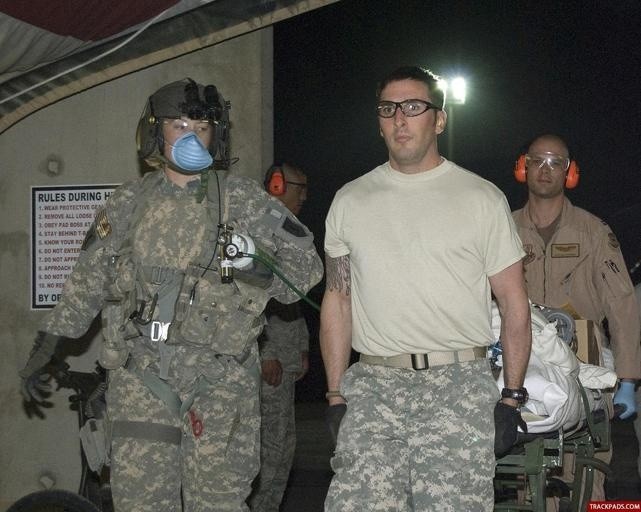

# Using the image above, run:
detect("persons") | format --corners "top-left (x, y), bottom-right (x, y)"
top-left (493, 132), bottom-right (641, 510)
top-left (16, 77), bottom-right (327, 512)
top-left (317, 62), bottom-right (536, 512)
top-left (248, 160), bottom-right (314, 512)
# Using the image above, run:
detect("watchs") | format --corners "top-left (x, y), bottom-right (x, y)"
top-left (501, 387), bottom-right (528, 404)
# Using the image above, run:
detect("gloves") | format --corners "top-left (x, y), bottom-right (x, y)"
top-left (494, 399), bottom-right (527, 454)
top-left (18, 341), bottom-right (61, 403)
top-left (613, 382), bottom-right (638, 420)
top-left (327, 403), bottom-right (347, 446)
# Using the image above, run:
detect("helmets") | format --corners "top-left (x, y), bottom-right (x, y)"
top-left (137, 79), bottom-right (231, 170)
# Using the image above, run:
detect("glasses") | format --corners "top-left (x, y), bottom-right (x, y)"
top-left (524, 153), bottom-right (569, 171)
top-left (170, 118), bottom-right (210, 134)
top-left (376, 99), bottom-right (441, 119)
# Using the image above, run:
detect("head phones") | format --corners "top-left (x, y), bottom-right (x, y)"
top-left (512, 128), bottom-right (582, 190)
top-left (269, 145), bottom-right (287, 196)
